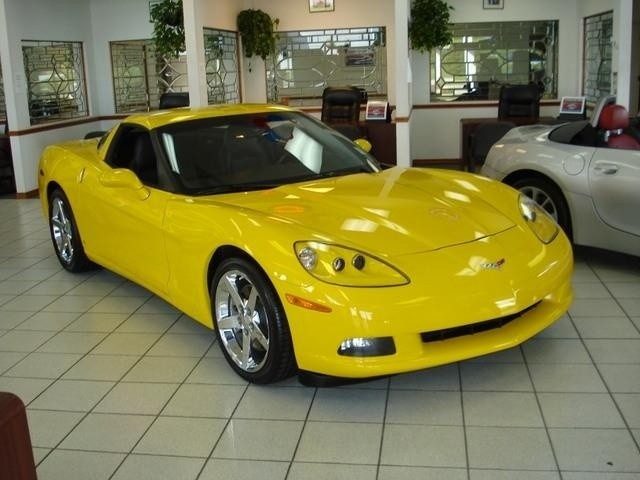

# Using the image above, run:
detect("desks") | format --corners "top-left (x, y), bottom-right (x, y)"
top-left (319, 120), bottom-right (396, 169)
top-left (460, 116), bottom-right (593, 173)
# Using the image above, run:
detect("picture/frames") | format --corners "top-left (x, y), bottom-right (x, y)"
top-left (309, 0), bottom-right (335, 13)
top-left (366, 100), bottom-right (388, 121)
top-left (559, 97), bottom-right (586, 114)
top-left (483, 0), bottom-right (505, 9)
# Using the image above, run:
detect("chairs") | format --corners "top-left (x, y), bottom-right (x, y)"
top-left (159, 92), bottom-right (189, 109)
top-left (464, 119), bottom-right (517, 174)
top-left (498, 83), bottom-right (540, 117)
top-left (330, 123), bottom-right (360, 140)
top-left (600, 104), bottom-right (638, 150)
top-left (321, 87), bottom-right (360, 122)
